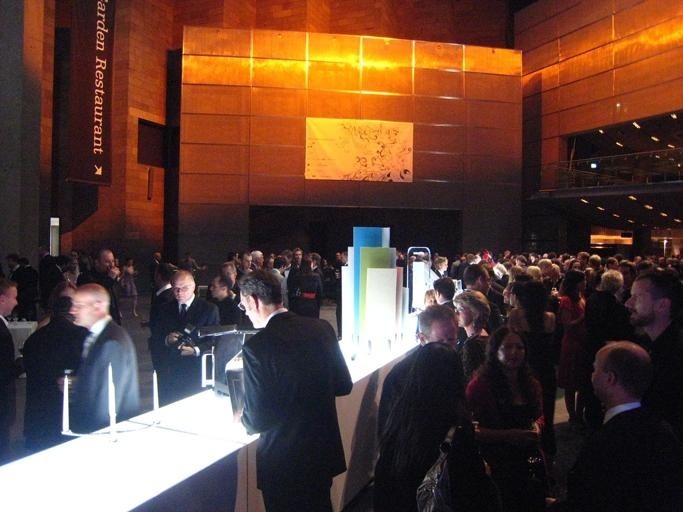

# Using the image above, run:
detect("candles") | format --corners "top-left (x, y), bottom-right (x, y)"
top-left (150, 366), bottom-right (162, 421)
top-left (106, 360), bottom-right (117, 434)
top-left (59, 370), bottom-right (71, 435)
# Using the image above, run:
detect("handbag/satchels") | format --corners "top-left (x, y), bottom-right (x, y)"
top-left (415, 418), bottom-right (460, 512)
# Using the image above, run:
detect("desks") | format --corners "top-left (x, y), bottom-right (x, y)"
top-left (8, 319), bottom-right (38, 379)
top-left (0, 416), bottom-right (252, 511)
top-left (141, 307), bottom-right (424, 511)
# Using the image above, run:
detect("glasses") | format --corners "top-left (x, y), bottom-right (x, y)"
top-left (238, 294), bottom-right (253, 312)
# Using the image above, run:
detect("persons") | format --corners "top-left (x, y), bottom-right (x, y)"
top-left (151, 248), bottom-right (335, 409)
top-left (336, 245), bottom-right (683, 512)
top-left (1, 246), bottom-right (141, 462)
top-left (238, 271), bottom-right (355, 511)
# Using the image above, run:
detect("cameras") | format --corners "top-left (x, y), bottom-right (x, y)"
top-left (176, 322), bottom-right (197, 355)
